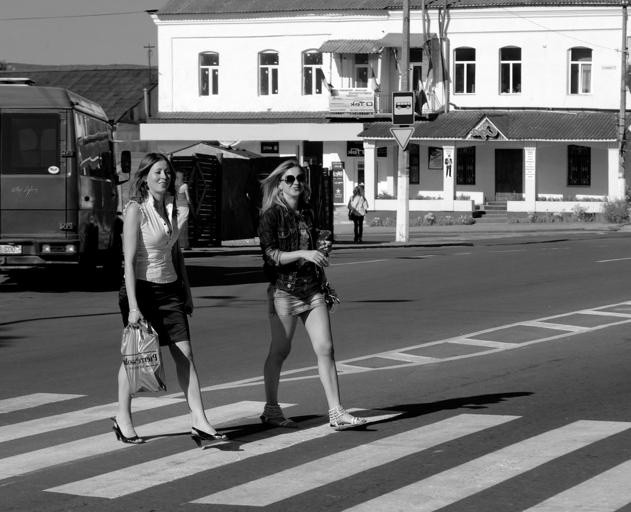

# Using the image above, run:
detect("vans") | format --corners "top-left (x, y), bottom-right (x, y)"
top-left (1, 85), bottom-right (123, 284)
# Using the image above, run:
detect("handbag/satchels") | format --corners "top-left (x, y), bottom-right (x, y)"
top-left (121, 320), bottom-right (168, 396)
top-left (320, 281), bottom-right (341, 311)
top-left (172, 246), bottom-right (194, 318)
top-left (348, 209), bottom-right (354, 220)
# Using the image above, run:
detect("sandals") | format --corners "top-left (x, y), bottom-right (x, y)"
top-left (260, 403), bottom-right (298, 428)
top-left (191, 427), bottom-right (228, 450)
top-left (109, 417), bottom-right (144, 444)
top-left (328, 405), bottom-right (369, 431)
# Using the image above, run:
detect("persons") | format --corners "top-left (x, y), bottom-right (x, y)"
top-left (173, 171), bottom-right (197, 251)
top-left (110, 150), bottom-right (229, 450)
top-left (347, 185), bottom-right (371, 244)
top-left (445, 155), bottom-right (452, 179)
top-left (258, 157), bottom-right (368, 431)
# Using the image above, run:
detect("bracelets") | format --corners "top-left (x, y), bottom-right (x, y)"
top-left (129, 308), bottom-right (142, 313)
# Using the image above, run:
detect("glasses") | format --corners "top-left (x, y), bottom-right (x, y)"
top-left (279, 173), bottom-right (305, 184)
